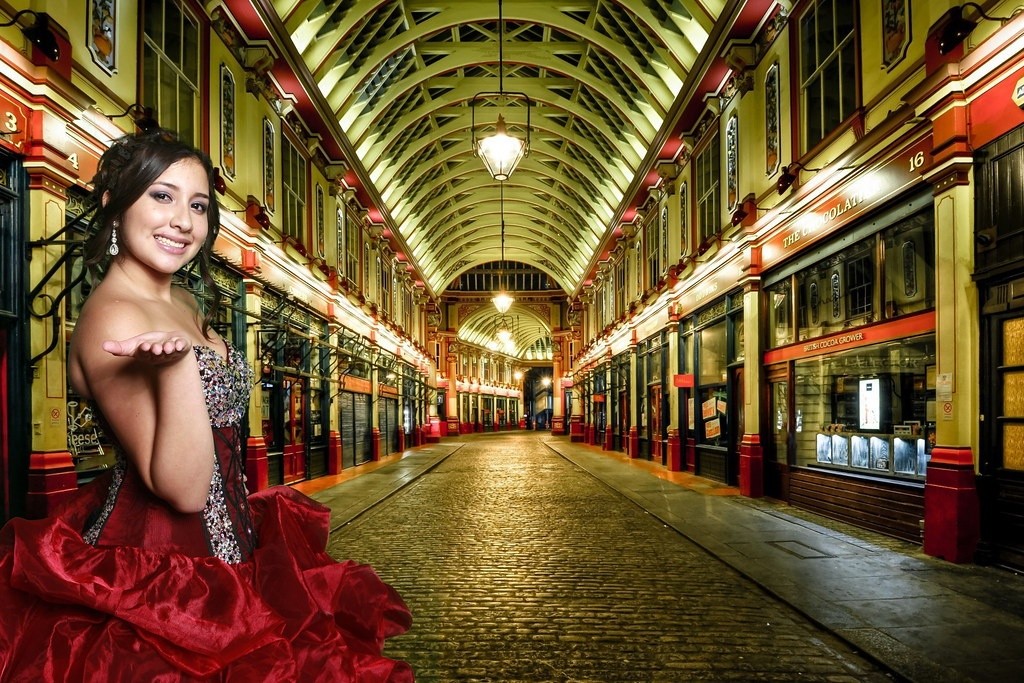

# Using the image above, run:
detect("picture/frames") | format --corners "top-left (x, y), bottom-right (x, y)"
top-left (810, 273), bottom-right (841, 324)
top-left (764, 62), bottom-right (781, 180)
top-left (680, 182), bottom-right (688, 259)
top-left (316, 182), bottom-right (326, 260)
top-left (87, 0), bottom-right (119, 76)
top-left (336, 207), bottom-right (344, 277)
top-left (727, 116), bottom-right (739, 214)
top-left (880, 0), bottom-right (913, 74)
top-left (602, 204), bottom-right (671, 329)
top-left (904, 239), bottom-right (918, 298)
top-left (219, 64), bottom-right (236, 182)
top-left (262, 116), bottom-right (275, 217)
top-left (364, 242), bottom-right (398, 322)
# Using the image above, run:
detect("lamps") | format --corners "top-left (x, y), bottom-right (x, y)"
top-left (303, 259), bottom-right (330, 277)
top-left (675, 256), bottom-right (704, 276)
top-left (573, 288), bottom-right (661, 360)
top-left (213, 167), bottom-right (226, 196)
top-left (470, 90), bottom-right (531, 182)
top-left (657, 273), bottom-right (682, 290)
top-left (233, 202), bottom-right (270, 230)
top-left (776, 162), bottom-right (821, 195)
top-left (939, 2), bottom-right (1011, 54)
top-left (333, 274), bottom-right (349, 292)
top-left (731, 202), bottom-right (770, 227)
top-left (698, 233), bottom-right (731, 256)
top-left (345, 289), bottom-right (435, 361)
top-left (106, 103), bottom-right (160, 134)
top-left (274, 235), bottom-right (308, 258)
top-left (0, 9), bottom-right (61, 62)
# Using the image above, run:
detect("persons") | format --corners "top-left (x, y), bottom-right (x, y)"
top-left (0, 107), bottom-right (415, 683)
